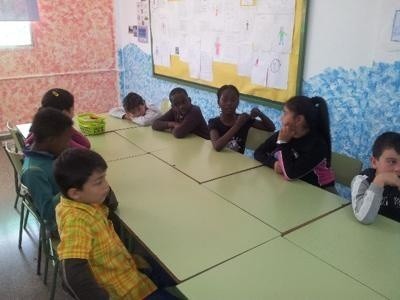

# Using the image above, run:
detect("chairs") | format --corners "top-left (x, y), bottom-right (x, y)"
top-left (246, 127), bottom-right (273, 151)
top-left (1, 120), bottom-right (45, 277)
top-left (50, 241), bottom-right (76, 300)
top-left (331, 151), bottom-right (362, 188)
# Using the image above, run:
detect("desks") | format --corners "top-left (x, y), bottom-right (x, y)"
top-left (74, 120), bottom-right (150, 162)
top-left (202, 165), bottom-right (351, 235)
top-left (101, 112), bottom-right (141, 131)
top-left (176, 236), bottom-right (389, 298)
top-left (116, 128), bottom-right (264, 184)
top-left (283, 202), bottom-right (400, 300)
top-left (106, 154), bottom-right (284, 284)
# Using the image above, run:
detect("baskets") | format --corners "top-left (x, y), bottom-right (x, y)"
top-left (78, 112), bottom-right (106, 135)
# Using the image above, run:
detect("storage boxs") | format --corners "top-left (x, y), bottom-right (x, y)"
top-left (77, 115), bottom-right (107, 136)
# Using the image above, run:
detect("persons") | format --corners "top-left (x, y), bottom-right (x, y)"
top-left (19, 106), bottom-right (76, 240)
top-left (349, 131), bottom-right (400, 226)
top-left (107, 92), bottom-right (164, 127)
top-left (18, 87), bottom-right (91, 151)
top-left (251, 95), bottom-right (340, 196)
top-left (152, 87), bottom-right (211, 140)
top-left (53, 146), bottom-right (182, 299)
top-left (207, 85), bottom-right (277, 156)
